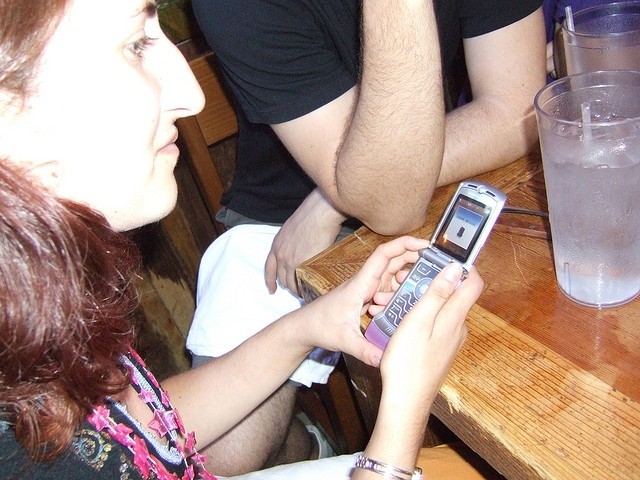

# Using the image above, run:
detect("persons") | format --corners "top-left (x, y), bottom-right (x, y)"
top-left (183, 0), bottom-right (549, 477)
top-left (1, 0), bottom-right (487, 479)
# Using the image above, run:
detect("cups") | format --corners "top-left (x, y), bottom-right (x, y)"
top-left (561, 1), bottom-right (640, 90)
top-left (534, 67), bottom-right (639, 308)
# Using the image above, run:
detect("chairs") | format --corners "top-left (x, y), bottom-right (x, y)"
top-left (174, 50), bottom-right (368, 454)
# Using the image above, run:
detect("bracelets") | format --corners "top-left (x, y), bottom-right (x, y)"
top-left (352, 455), bottom-right (424, 480)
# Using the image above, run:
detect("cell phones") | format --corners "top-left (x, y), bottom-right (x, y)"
top-left (365, 180), bottom-right (508, 353)
